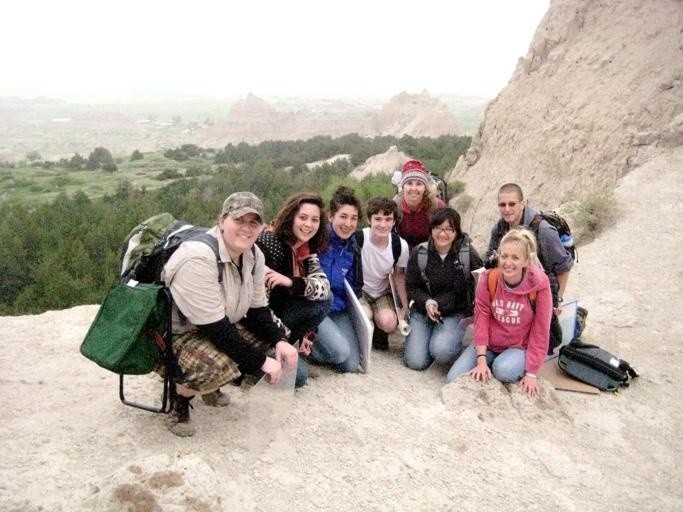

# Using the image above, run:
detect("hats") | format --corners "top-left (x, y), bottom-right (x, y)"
top-left (221, 190), bottom-right (265, 226)
top-left (391, 159), bottom-right (434, 201)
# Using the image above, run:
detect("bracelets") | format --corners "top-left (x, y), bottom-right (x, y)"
top-left (476, 354), bottom-right (488, 360)
top-left (525, 372), bottom-right (537, 379)
top-left (556, 296), bottom-right (564, 302)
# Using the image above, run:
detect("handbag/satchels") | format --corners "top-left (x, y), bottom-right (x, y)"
top-left (77, 280), bottom-right (176, 375)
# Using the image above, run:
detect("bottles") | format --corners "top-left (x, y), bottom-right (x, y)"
top-left (560, 235), bottom-right (573, 248)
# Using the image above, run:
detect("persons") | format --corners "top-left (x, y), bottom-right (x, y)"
top-left (151, 191), bottom-right (300, 438)
top-left (255, 190), bottom-right (350, 388)
top-left (401, 207), bottom-right (483, 370)
top-left (354, 194), bottom-right (410, 352)
top-left (391, 161), bottom-right (448, 247)
top-left (484, 183), bottom-right (574, 355)
top-left (445, 225), bottom-right (554, 398)
top-left (313, 186), bottom-right (365, 374)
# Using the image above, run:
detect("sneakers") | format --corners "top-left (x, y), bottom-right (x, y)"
top-left (164, 401), bottom-right (194, 438)
top-left (371, 327), bottom-right (390, 354)
top-left (201, 389), bottom-right (232, 408)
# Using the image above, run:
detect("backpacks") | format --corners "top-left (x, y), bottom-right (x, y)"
top-left (114, 212), bottom-right (224, 306)
top-left (531, 209), bottom-right (578, 271)
top-left (428, 175), bottom-right (450, 205)
top-left (558, 342), bottom-right (639, 396)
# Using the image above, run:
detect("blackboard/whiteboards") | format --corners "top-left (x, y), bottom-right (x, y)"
top-left (544, 300), bottom-right (577, 363)
